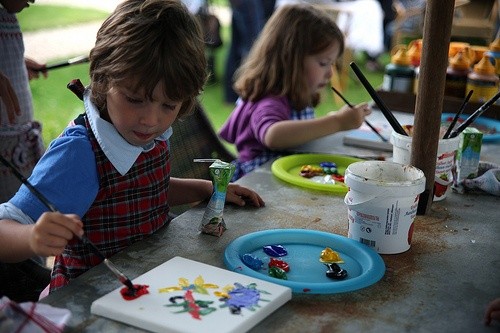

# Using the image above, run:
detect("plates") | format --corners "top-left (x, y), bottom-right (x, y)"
top-left (224, 228), bottom-right (386, 293)
top-left (271, 153), bottom-right (366, 193)
top-left (441, 114), bottom-right (500, 143)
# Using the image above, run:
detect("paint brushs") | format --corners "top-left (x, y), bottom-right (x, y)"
top-left (349, 62), bottom-right (500, 139)
top-left (0, 152), bottom-right (134, 296)
top-left (30, 54), bottom-right (89, 74)
top-left (331, 86), bottom-right (387, 142)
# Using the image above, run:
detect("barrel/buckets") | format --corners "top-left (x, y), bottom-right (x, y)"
top-left (344, 160), bottom-right (426, 254)
top-left (390, 125), bottom-right (460, 202)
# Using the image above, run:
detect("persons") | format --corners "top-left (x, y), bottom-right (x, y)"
top-left (0, 0), bottom-right (45, 204)
top-left (0, 0), bottom-right (266, 297)
top-left (218, 3), bottom-right (373, 182)
top-left (178, 0), bottom-right (427, 103)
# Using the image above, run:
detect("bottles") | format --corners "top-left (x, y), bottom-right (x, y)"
top-left (382, 39), bottom-right (500, 104)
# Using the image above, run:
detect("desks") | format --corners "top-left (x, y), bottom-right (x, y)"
top-left (37, 110), bottom-right (500, 333)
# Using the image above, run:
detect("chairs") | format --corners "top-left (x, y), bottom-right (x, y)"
top-left (168, 101), bottom-right (237, 221)
